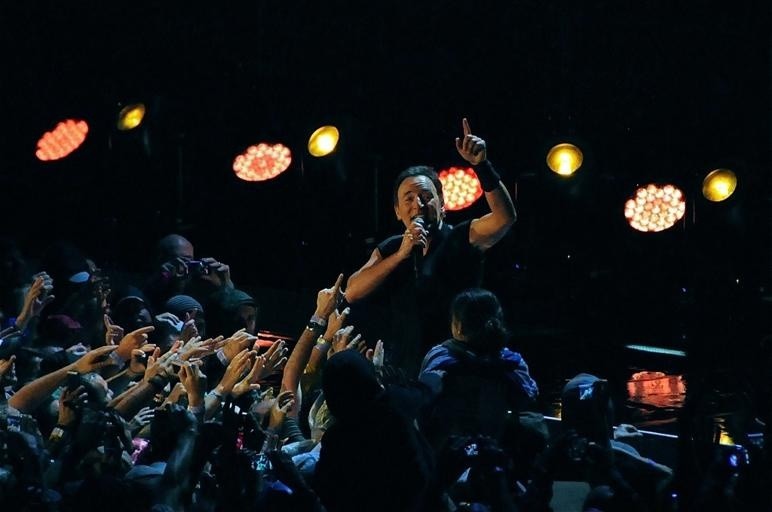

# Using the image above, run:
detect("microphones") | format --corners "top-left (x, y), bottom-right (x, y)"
top-left (413, 217), bottom-right (425, 279)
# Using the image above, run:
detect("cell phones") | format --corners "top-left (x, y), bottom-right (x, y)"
top-left (66, 371), bottom-right (80, 393)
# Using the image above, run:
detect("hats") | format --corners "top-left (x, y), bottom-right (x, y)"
top-left (39, 313), bottom-right (79, 335)
top-left (167, 294), bottom-right (204, 314)
top-left (113, 284), bottom-right (144, 309)
top-left (213, 290), bottom-right (256, 311)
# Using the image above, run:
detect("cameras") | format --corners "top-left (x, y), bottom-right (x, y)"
top-left (178, 259), bottom-right (208, 278)
top-left (172, 362), bottom-right (183, 377)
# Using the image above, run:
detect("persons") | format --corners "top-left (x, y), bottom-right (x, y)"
top-left (342, 117), bottom-right (519, 374)
top-left (147, 234), bottom-right (232, 290)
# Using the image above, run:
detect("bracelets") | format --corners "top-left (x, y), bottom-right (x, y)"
top-left (472, 160), bottom-right (501, 191)
